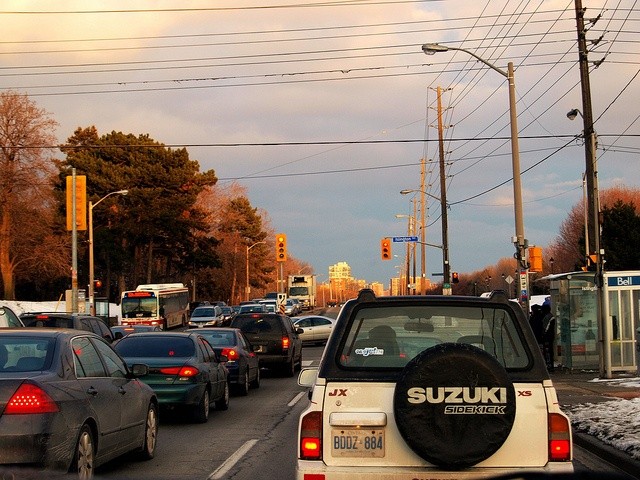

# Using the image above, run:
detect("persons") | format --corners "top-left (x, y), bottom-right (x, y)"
top-left (539, 304), bottom-right (561, 368)
top-left (526, 301), bottom-right (541, 337)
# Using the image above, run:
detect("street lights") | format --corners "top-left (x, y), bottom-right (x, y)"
top-left (424, 42), bottom-right (534, 312)
top-left (514, 270), bottom-right (519, 298)
top-left (400, 188), bottom-right (450, 293)
top-left (485, 275), bottom-right (492, 292)
top-left (396, 214), bottom-right (426, 295)
top-left (396, 271), bottom-right (401, 295)
top-left (393, 265), bottom-right (405, 294)
top-left (565, 108), bottom-right (603, 267)
top-left (89, 188), bottom-right (130, 317)
top-left (549, 257), bottom-right (555, 273)
top-left (393, 254), bottom-right (406, 295)
top-left (501, 273), bottom-right (505, 291)
top-left (246, 240), bottom-right (266, 301)
top-left (474, 281), bottom-right (478, 296)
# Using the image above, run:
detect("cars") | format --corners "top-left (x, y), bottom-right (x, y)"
top-left (210, 301), bottom-right (226, 306)
top-left (1, 328), bottom-right (160, 480)
top-left (111, 325), bottom-right (165, 340)
top-left (190, 299), bottom-right (210, 312)
top-left (291, 299), bottom-right (302, 313)
top-left (239, 300), bottom-right (277, 312)
top-left (221, 306), bottom-right (237, 325)
top-left (285, 299), bottom-right (295, 314)
top-left (292, 316), bottom-right (337, 345)
top-left (113, 331), bottom-right (230, 423)
top-left (189, 304), bottom-right (224, 327)
top-left (183, 328), bottom-right (262, 396)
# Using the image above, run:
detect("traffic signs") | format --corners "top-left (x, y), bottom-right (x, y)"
top-left (393, 236), bottom-right (418, 242)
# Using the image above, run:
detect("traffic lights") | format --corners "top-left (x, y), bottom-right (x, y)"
top-left (277, 233), bottom-right (287, 262)
top-left (380, 239), bottom-right (391, 260)
top-left (452, 272), bottom-right (459, 283)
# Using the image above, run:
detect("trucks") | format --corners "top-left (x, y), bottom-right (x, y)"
top-left (287, 275), bottom-right (316, 310)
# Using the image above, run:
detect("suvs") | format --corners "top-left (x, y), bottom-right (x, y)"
top-left (296, 296), bottom-right (574, 479)
top-left (16, 312), bottom-right (114, 344)
top-left (227, 313), bottom-right (303, 377)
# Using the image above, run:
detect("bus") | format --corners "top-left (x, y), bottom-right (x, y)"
top-left (121, 281), bottom-right (190, 331)
